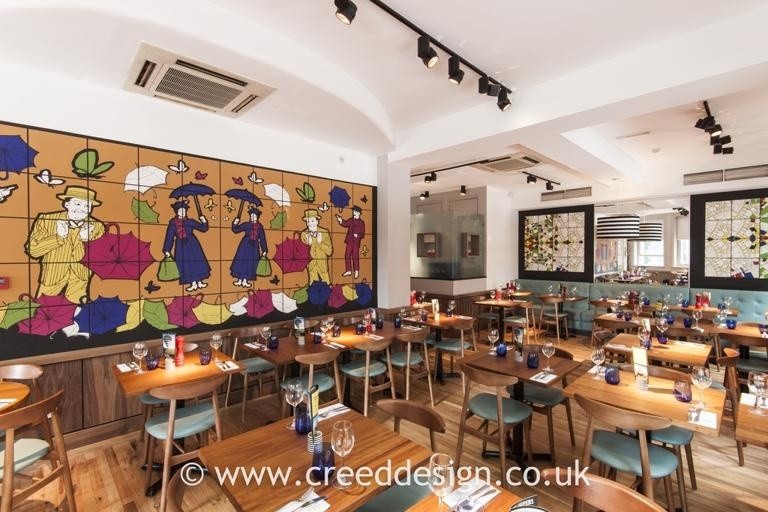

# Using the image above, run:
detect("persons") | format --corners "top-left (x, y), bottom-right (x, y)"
top-left (163, 202), bottom-right (212, 292)
top-left (300, 209), bottom-right (333, 286)
top-left (229, 208), bottom-right (268, 288)
top-left (24, 185), bottom-right (107, 305)
top-left (335, 206), bottom-right (365, 279)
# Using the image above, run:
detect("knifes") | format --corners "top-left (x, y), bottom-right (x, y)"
top-left (290, 495), bottom-right (328, 511)
top-left (449, 485), bottom-right (489, 511)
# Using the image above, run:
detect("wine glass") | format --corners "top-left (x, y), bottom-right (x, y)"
top-left (330, 421), bottom-right (355, 489)
top-left (610, 290), bottom-right (767, 351)
top-left (490, 279), bottom-right (577, 302)
top-left (285, 379), bottom-right (304, 431)
top-left (488, 327), bottom-right (712, 411)
top-left (427, 453), bottom-right (455, 512)
top-left (456, 496), bottom-right (484, 512)
top-left (747, 371), bottom-right (768, 415)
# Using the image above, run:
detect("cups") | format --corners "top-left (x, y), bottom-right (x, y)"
top-left (497, 342), bottom-right (692, 403)
top-left (312, 442), bottom-right (335, 481)
top-left (295, 404), bottom-right (311, 436)
top-left (602, 295), bottom-right (768, 351)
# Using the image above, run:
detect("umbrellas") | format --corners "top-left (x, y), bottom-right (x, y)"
top-left (273, 231), bottom-right (313, 274)
top-left (123, 162), bottom-right (171, 199)
top-left (80, 222), bottom-right (156, 280)
top-left (0, 135), bottom-right (39, 180)
top-left (328, 185), bottom-right (351, 213)
top-left (263, 183), bottom-right (291, 209)
top-left (224, 188), bottom-right (263, 219)
top-left (269, 211), bottom-right (287, 230)
top-left (131, 197), bottom-right (160, 224)
top-left (169, 182), bottom-right (216, 219)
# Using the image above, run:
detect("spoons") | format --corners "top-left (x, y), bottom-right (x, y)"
top-left (462, 488), bottom-right (496, 510)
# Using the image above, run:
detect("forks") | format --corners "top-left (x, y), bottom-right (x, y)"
top-left (269, 485), bottom-right (315, 512)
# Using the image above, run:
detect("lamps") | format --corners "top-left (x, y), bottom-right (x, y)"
top-left (418, 36), bottom-right (439, 68)
top-left (695, 101), bottom-right (734, 154)
top-left (424, 171), bottom-right (437, 183)
top-left (334, 0), bottom-right (357, 25)
top-left (460, 185), bottom-right (466, 196)
top-left (496, 88), bottom-right (511, 112)
top-left (527, 174), bottom-right (537, 183)
top-left (447, 56), bottom-right (464, 85)
top-left (419, 190), bottom-right (429, 200)
top-left (478, 76), bottom-right (500, 97)
top-left (546, 180), bottom-right (553, 190)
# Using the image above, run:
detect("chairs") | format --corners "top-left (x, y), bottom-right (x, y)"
top-left (0, 286), bottom-right (768, 511)
